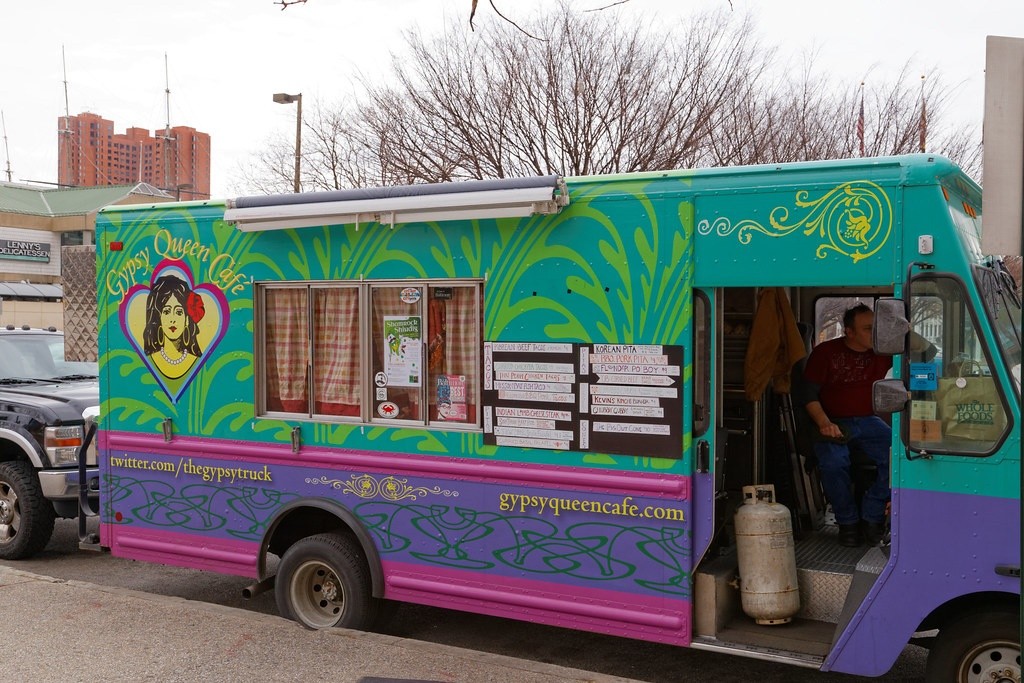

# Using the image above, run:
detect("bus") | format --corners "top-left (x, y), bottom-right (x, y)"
top-left (75, 153), bottom-right (1023, 683)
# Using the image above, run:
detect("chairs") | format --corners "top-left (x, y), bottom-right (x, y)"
top-left (795, 319), bottom-right (876, 504)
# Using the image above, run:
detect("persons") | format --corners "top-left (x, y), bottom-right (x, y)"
top-left (801, 303), bottom-right (937, 548)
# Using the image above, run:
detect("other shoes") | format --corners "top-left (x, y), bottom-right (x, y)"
top-left (861, 514), bottom-right (886, 546)
top-left (837, 522), bottom-right (864, 546)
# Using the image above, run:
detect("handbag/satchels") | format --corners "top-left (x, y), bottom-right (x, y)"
top-left (939, 360), bottom-right (1007, 441)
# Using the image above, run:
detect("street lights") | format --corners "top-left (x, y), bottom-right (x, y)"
top-left (272, 92), bottom-right (305, 194)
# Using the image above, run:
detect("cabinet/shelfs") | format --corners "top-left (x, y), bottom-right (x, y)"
top-left (724, 311), bottom-right (754, 435)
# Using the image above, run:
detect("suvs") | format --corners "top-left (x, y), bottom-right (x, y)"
top-left (0, 324), bottom-right (99, 561)
